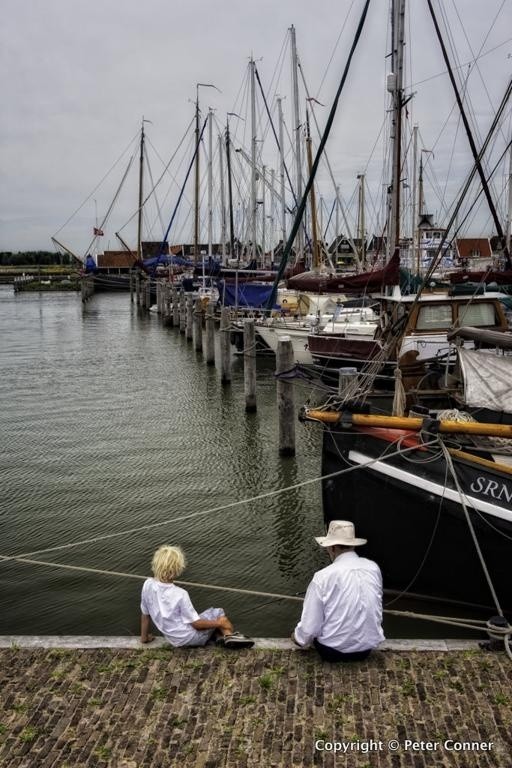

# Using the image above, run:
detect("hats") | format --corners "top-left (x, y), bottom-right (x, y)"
top-left (315, 520), bottom-right (367, 547)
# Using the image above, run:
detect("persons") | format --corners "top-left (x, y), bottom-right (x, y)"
top-left (138, 546), bottom-right (256, 650)
top-left (290, 519), bottom-right (386, 664)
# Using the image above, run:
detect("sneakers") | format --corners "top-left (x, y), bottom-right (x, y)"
top-left (216, 631), bottom-right (254, 648)
